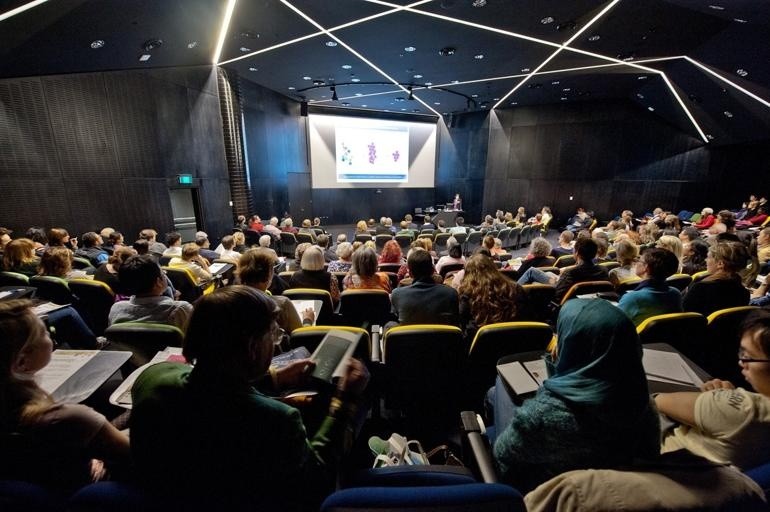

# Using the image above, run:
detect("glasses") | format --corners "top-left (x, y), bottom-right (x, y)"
top-left (261, 329), bottom-right (286, 345)
top-left (738, 351), bottom-right (769, 365)
top-left (636, 259), bottom-right (647, 265)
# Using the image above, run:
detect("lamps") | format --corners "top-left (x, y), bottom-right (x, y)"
top-left (300, 95), bottom-right (308, 116)
top-left (331, 85), bottom-right (339, 101)
top-left (407, 87), bottom-right (415, 100)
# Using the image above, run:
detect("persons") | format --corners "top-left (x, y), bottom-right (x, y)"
top-left (650, 318), bottom-right (769, 470)
top-left (2, 191), bottom-right (769, 361)
top-left (0, 298), bottom-right (130, 511)
top-left (491, 298), bottom-right (662, 496)
top-left (129, 283), bottom-right (371, 511)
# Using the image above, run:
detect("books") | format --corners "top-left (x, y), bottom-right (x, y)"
top-left (642, 346), bottom-right (704, 391)
top-left (305, 325), bottom-right (368, 383)
